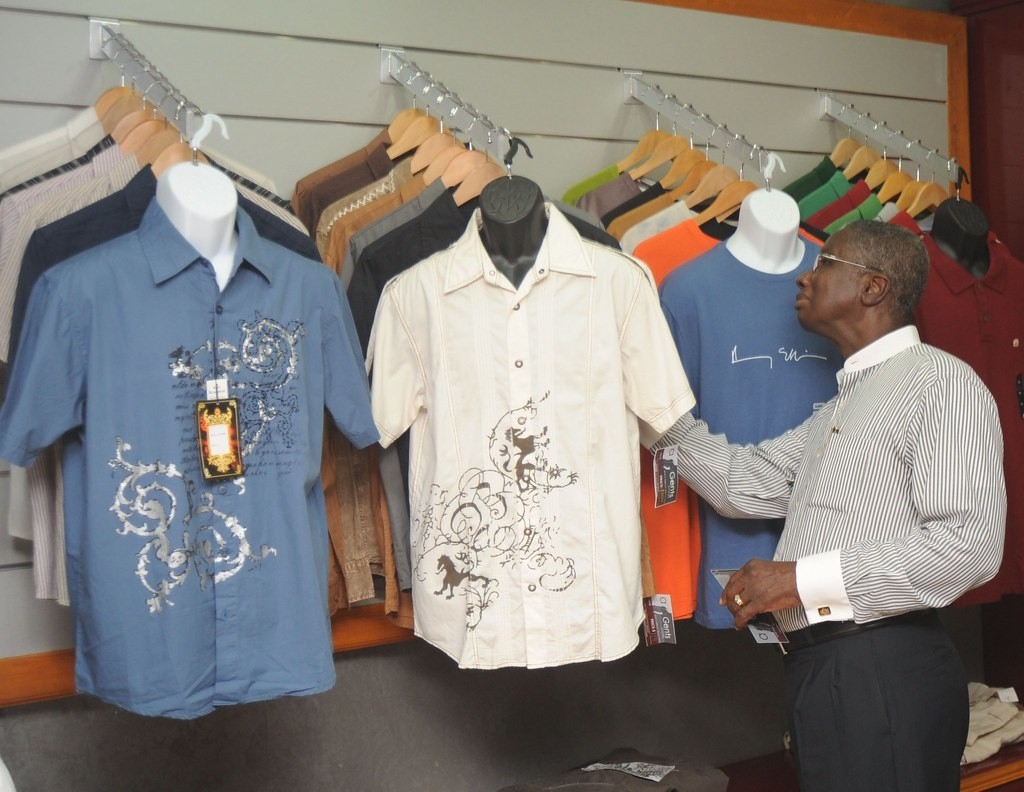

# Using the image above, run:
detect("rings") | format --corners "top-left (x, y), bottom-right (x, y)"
top-left (734, 595), bottom-right (744, 607)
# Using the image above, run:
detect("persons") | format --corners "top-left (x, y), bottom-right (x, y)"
top-left (651, 221), bottom-right (1005, 791)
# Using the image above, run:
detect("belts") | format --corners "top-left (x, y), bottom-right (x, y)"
top-left (778, 608), bottom-right (938, 654)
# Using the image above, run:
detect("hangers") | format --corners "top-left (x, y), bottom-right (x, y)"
top-left (92, 32), bottom-right (242, 294)
top-left (834, 98), bottom-right (991, 280)
top-left (383, 61), bottom-right (549, 288)
top-left (615, 74), bottom-right (808, 274)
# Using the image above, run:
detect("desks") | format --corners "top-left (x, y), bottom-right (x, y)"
top-left (724, 742), bottom-right (1024, 792)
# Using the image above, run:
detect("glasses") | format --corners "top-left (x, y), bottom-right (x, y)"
top-left (811, 253), bottom-right (882, 275)
